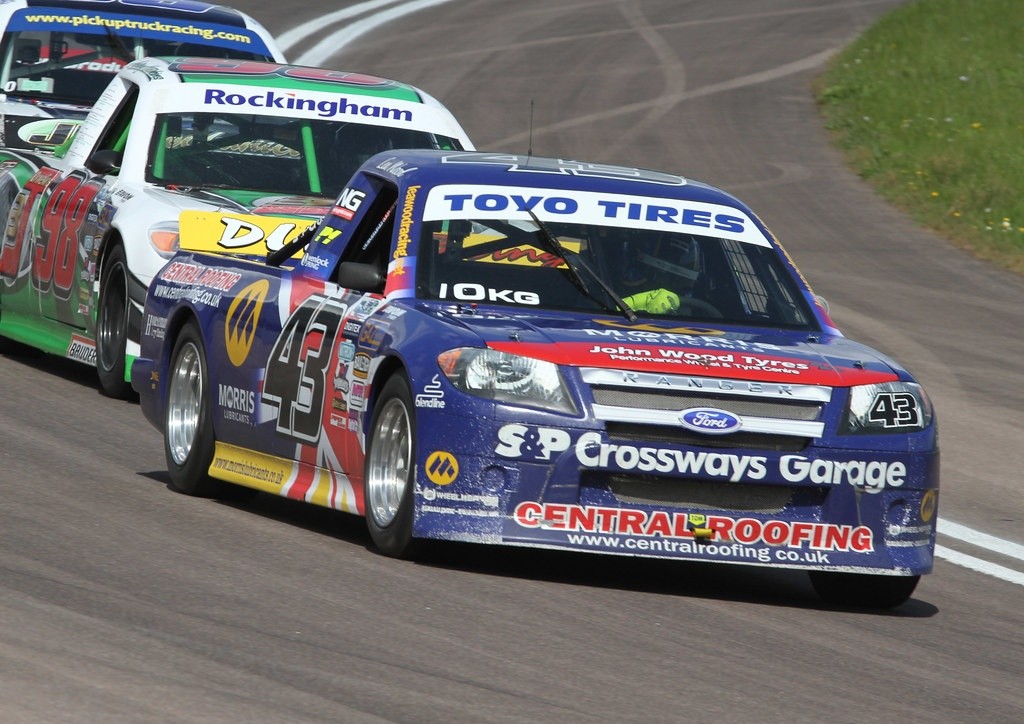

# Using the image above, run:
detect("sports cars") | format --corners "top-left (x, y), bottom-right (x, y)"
top-left (130, 149), bottom-right (941, 609)
top-left (0, 1), bottom-right (289, 162)
top-left (0, 55), bottom-right (477, 400)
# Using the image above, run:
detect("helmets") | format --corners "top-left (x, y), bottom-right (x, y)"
top-left (332, 123), bottom-right (394, 178)
top-left (626, 231), bottom-right (704, 296)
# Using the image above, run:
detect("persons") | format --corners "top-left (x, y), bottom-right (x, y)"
top-left (324, 123), bottom-right (393, 200)
top-left (584, 227), bottom-right (702, 317)
